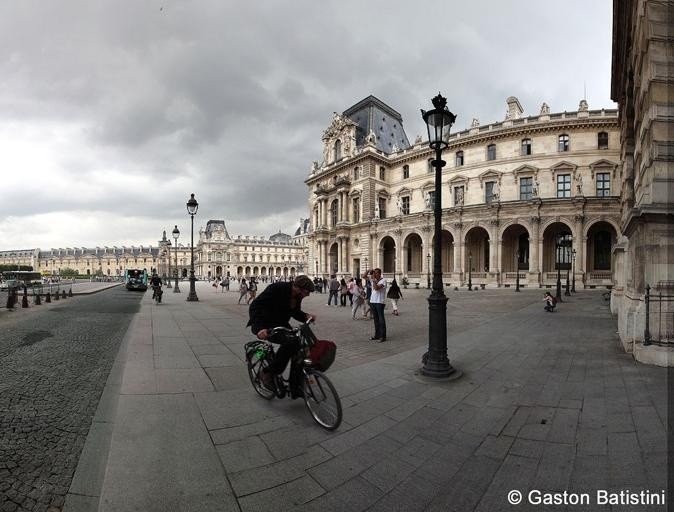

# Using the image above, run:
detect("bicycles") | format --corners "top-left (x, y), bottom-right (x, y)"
top-left (242, 314), bottom-right (342, 433)
top-left (600, 290), bottom-right (614, 307)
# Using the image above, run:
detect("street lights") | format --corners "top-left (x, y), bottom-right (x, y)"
top-left (554, 231), bottom-right (577, 304)
top-left (362, 246), bottom-right (522, 295)
top-left (417, 92), bottom-right (459, 379)
top-left (156, 238), bottom-right (173, 289)
top-left (171, 225), bottom-right (182, 294)
top-left (184, 192), bottom-right (202, 303)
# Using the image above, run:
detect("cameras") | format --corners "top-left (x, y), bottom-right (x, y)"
top-left (370, 270), bottom-right (375, 275)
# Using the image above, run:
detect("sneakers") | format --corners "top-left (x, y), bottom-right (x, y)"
top-left (369, 337), bottom-right (386, 342)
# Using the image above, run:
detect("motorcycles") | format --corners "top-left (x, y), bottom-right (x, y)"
top-left (150, 284), bottom-right (163, 305)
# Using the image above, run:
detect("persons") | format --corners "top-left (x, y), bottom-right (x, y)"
top-left (211, 270), bottom-right (404, 321)
top-left (542, 291), bottom-right (556, 311)
top-left (245, 275), bottom-right (316, 400)
top-left (363, 268), bottom-right (387, 342)
top-left (149, 273), bottom-right (163, 299)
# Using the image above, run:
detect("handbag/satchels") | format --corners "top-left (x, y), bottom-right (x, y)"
top-left (308, 340), bottom-right (337, 372)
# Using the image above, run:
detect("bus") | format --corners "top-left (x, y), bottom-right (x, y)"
top-left (124, 268), bottom-right (148, 291)
top-left (0, 271), bottom-right (42, 289)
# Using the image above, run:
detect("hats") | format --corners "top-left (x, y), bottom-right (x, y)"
top-left (294, 275), bottom-right (315, 292)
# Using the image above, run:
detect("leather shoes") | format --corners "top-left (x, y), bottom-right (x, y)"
top-left (258, 371), bottom-right (275, 391)
top-left (291, 391), bottom-right (312, 400)
